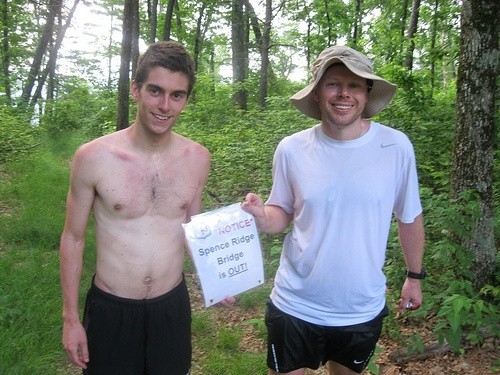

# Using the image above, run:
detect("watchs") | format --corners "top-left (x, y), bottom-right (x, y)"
top-left (405, 268), bottom-right (427, 279)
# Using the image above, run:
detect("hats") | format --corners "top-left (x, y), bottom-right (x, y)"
top-left (289, 46), bottom-right (397, 120)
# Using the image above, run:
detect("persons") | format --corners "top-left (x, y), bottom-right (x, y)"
top-left (241, 45), bottom-right (427, 375)
top-left (59, 42), bottom-right (236, 375)
